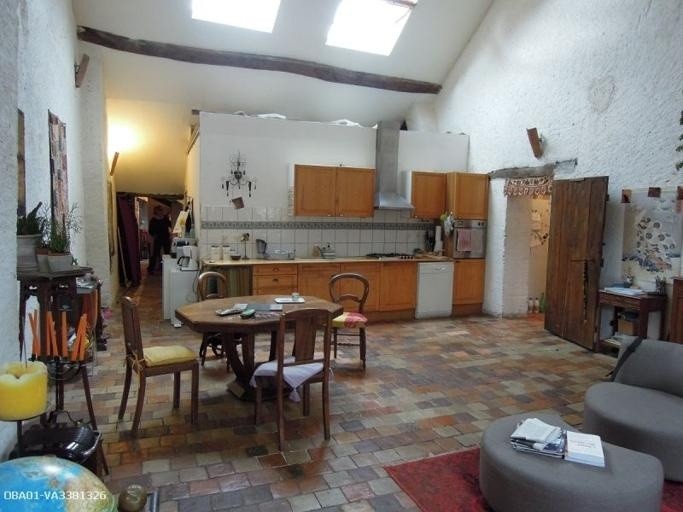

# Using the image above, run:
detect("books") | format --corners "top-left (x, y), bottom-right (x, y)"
top-left (564, 429), bottom-right (605, 468)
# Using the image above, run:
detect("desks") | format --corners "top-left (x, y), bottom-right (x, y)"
top-left (593, 285), bottom-right (668, 360)
top-left (14, 265), bottom-right (109, 478)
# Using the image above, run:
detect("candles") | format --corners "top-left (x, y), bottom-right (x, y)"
top-left (0, 363), bottom-right (47, 420)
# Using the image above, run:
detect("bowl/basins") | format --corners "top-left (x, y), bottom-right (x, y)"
top-left (230, 255), bottom-right (241, 260)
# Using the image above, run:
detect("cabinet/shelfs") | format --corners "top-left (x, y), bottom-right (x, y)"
top-left (378, 262), bottom-right (417, 312)
top-left (444, 172), bottom-right (489, 219)
top-left (250, 265), bottom-right (297, 295)
top-left (452, 260), bottom-right (484, 305)
top-left (404, 170), bottom-right (446, 221)
top-left (297, 262), bottom-right (378, 312)
top-left (287, 164), bottom-right (376, 218)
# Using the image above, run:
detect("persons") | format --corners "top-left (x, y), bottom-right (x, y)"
top-left (147, 207), bottom-right (173, 275)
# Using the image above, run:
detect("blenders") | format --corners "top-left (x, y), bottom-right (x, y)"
top-left (254, 238), bottom-right (268, 260)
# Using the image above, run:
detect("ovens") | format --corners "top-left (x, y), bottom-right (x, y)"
top-left (445, 220), bottom-right (487, 259)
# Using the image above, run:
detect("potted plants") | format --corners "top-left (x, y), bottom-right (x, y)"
top-left (16, 200), bottom-right (84, 271)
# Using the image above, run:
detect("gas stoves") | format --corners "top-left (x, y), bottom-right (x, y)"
top-left (367, 252), bottom-right (418, 262)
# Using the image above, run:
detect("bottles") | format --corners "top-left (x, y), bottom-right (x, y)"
top-left (527, 291), bottom-right (544, 313)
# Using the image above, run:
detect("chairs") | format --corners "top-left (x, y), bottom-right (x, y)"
top-left (580, 337), bottom-right (683, 484)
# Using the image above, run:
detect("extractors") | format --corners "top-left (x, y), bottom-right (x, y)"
top-left (374, 116), bottom-right (417, 212)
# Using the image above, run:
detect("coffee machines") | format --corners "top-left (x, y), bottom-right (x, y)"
top-left (177, 246), bottom-right (199, 271)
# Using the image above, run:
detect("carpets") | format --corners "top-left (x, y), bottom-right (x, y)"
top-left (381, 444), bottom-right (682, 512)
